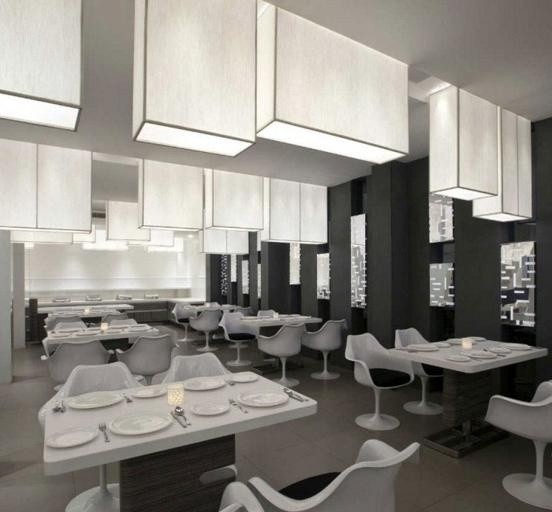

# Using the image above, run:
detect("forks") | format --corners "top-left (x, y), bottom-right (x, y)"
top-left (99, 422), bottom-right (110, 442)
top-left (229, 398), bottom-right (247, 414)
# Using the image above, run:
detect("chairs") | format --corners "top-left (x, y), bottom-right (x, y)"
top-left (172, 302), bottom-right (276, 367)
top-left (162, 353), bottom-right (231, 383)
top-left (115, 334), bottom-right (180, 385)
top-left (302, 319), bottom-right (347, 381)
top-left (38, 361), bottom-right (145, 512)
top-left (40, 339), bottom-right (115, 393)
top-left (394, 328), bottom-right (443, 417)
top-left (247, 439), bottom-right (421, 512)
top-left (43, 308), bottom-right (138, 334)
top-left (258, 322), bottom-right (306, 388)
top-left (345, 332), bottom-right (415, 432)
top-left (483, 381), bottom-right (552, 511)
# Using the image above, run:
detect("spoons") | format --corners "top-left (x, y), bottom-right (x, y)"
top-left (224, 378), bottom-right (234, 385)
top-left (176, 406), bottom-right (190, 425)
top-left (53, 401), bottom-right (59, 412)
top-left (284, 387), bottom-right (302, 401)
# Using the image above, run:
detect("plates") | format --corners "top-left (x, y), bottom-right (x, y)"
top-left (489, 346), bottom-right (512, 353)
top-left (88, 327), bottom-right (100, 330)
top-left (468, 336), bottom-right (485, 341)
top-left (133, 385), bottom-right (167, 398)
top-left (263, 319), bottom-right (282, 321)
top-left (128, 328), bottom-right (153, 331)
top-left (407, 343), bottom-right (438, 351)
top-left (506, 342), bottom-right (532, 350)
top-left (184, 377), bottom-right (225, 390)
top-left (191, 400), bottom-right (230, 415)
top-left (111, 325), bottom-right (129, 328)
top-left (105, 329), bottom-right (123, 332)
top-left (448, 339), bottom-right (476, 344)
top-left (76, 332), bottom-right (97, 336)
top-left (447, 355), bottom-right (470, 362)
top-left (467, 352), bottom-right (497, 359)
top-left (130, 324), bottom-right (145, 327)
top-left (240, 316), bottom-right (259, 319)
top-left (68, 392), bottom-right (122, 409)
top-left (280, 316), bottom-right (313, 320)
top-left (45, 424), bottom-right (98, 449)
top-left (61, 329), bottom-right (80, 332)
top-left (52, 333), bottom-right (72, 337)
top-left (237, 389), bottom-right (288, 407)
top-left (109, 409), bottom-right (173, 436)
top-left (434, 341), bottom-right (450, 348)
top-left (229, 371), bottom-right (258, 382)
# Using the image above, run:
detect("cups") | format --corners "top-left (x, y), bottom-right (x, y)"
top-left (463, 338), bottom-right (473, 350)
top-left (273, 312), bottom-right (278, 319)
top-left (167, 384), bottom-right (184, 406)
top-left (101, 323), bottom-right (108, 332)
